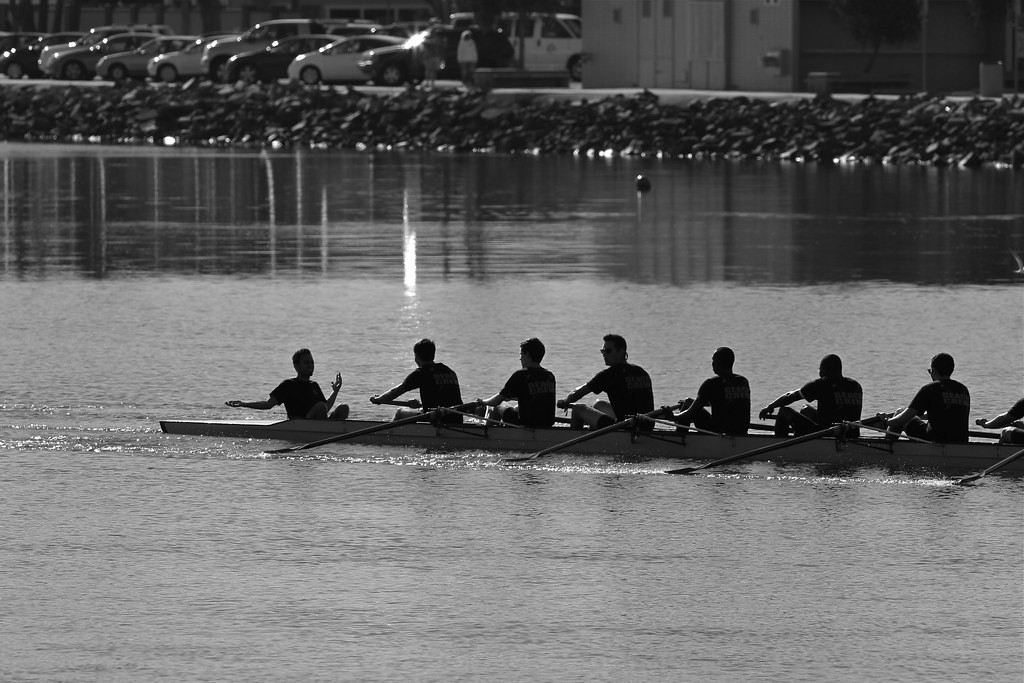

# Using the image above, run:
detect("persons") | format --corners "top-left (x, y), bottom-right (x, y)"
top-left (976, 398), bottom-right (1024, 445)
top-left (660, 347), bottom-right (750, 435)
top-left (563, 334), bottom-right (655, 431)
top-left (458, 31), bottom-right (477, 86)
top-left (224, 348), bottom-right (349, 419)
top-left (475, 337), bottom-right (556, 427)
top-left (759, 354), bottom-right (863, 436)
top-left (876, 353), bottom-right (971, 443)
top-left (371, 339), bottom-right (463, 424)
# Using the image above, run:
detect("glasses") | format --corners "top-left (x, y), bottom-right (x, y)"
top-left (600, 349), bottom-right (620, 354)
top-left (928, 368), bottom-right (938, 375)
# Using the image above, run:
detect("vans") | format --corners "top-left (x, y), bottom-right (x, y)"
top-left (452, 13), bottom-right (585, 82)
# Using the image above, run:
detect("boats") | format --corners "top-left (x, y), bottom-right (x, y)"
top-left (160, 421), bottom-right (1024, 475)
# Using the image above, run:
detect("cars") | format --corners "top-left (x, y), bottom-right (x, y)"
top-left (0, 19), bottom-right (455, 85)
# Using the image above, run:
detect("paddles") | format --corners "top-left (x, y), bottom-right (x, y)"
top-left (370, 397), bottom-right (571, 424)
top-left (955, 448), bottom-right (1024, 485)
top-left (664, 411), bottom-right (895, 476)
top-left (558, 401), bottom-right (796, 434)
top-left (501, 399), bottom-right (684, 462)
top-left (264, 400), bottom-right (482, 455)
top-left (763, 414), bottom-right (1002, 440)
